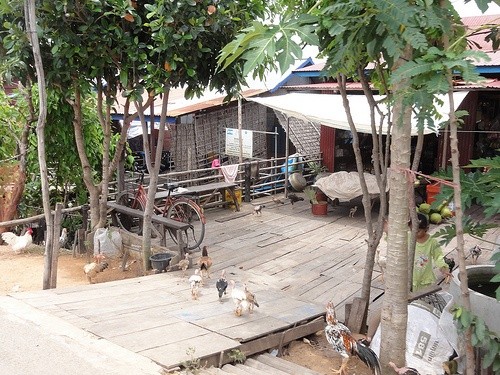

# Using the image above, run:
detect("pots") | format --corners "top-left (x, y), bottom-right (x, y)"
top-left (287, 172), bottom-right (307, 192)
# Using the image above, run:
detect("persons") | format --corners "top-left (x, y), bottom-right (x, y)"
top-left (407, 212), bottom-right (452, 297)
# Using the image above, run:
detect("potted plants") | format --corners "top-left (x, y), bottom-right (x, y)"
top-left (302, 187), bottom-right (327, 215)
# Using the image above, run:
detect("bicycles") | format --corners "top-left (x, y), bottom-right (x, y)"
top-left (113, 163), bottom-right (205, 257)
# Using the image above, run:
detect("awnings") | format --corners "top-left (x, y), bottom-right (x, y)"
top-left (249, 91), bottom-right (468, 134)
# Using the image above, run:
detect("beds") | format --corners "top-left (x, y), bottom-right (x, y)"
top-left (313, 170), bottom-right (392, 207)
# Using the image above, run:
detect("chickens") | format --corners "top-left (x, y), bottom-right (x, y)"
top-left (270, 196), bottom-right (284, 207)
top-left (251, 205), bottom-right (265, 216)
top-left (443, 256), bottom-right (455, 273)
top-left (83, 254), bottom-right (109, 284)
top-left (42, 228), bottom-right (68, 249)
top-left (216, 269), bottom-right (228, 301)
top-left (176, 253), bottom-right (189, 276)
top-left (1, 227), bottom-right (33, 255)
top-left (234, 283), bottom-right (260, 317)
top-left (386, 361), bottom-right (421, 375)
top-left (468, 244), bottom-right (482, 264)
top-left (348, 206), bottom-right (358, 219)
top-left (324, 301), bottom-right (383, 375)
top-left (198, 245), bottom-right (213, 283)
top-left (286, 188), bottom-right (304, 207)
top-left (188, 268), bottom-right (202, 299)
